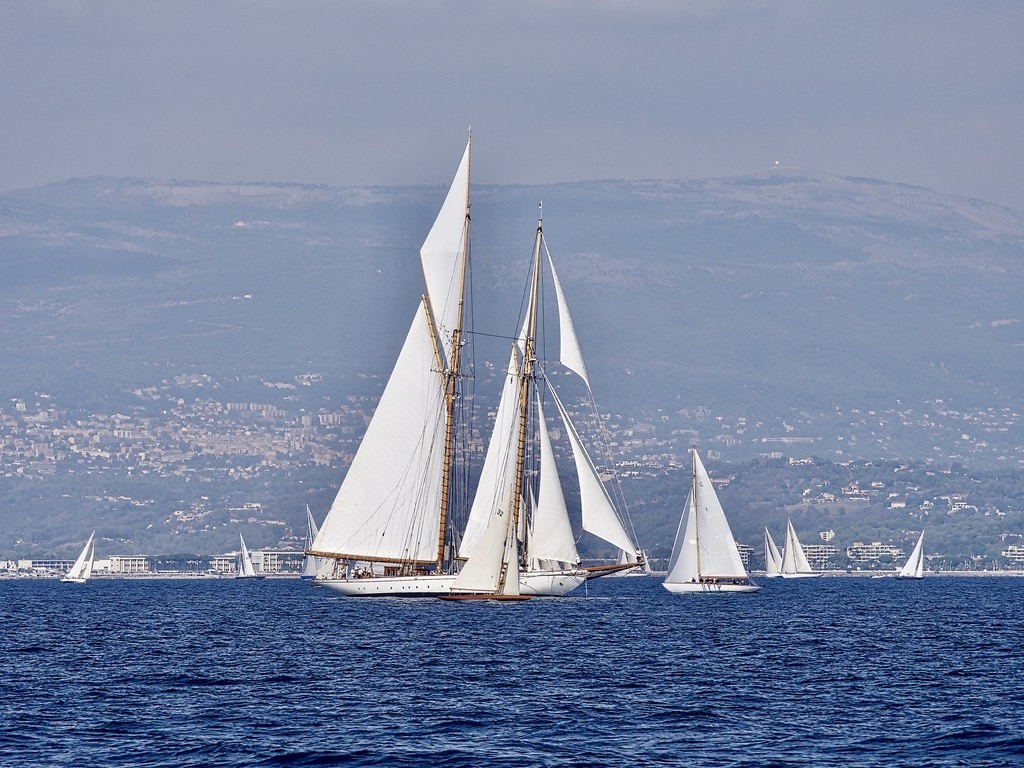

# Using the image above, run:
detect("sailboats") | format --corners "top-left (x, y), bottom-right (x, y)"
top-left (235, 534), bottom-right (267, 580)
top-left (58, 528), bottom-right (95, 584)
top-left (781, 517), bottom-right (825, 578)
top-left (763, 526), bottom-right (784, 577)
top-left (293, 122), bottom-right (652, 601)
top-left (895, 530), bottom-right (929, 580)
top-left (658, 444), bottom-right (763, 594)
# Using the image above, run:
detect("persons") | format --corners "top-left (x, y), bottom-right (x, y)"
top-left (692, 577), bottom-right (695, 582)
top-left (321, 574), bottom-right (327, 580)
top-left (350, 565), bottom-right (367, 579)
top-left (699, 577), bottom-right (703, 584)
top-left (635, 547), bottom-right (641, 562)
top-left (340, 566), bottom-right (346, 579)
top-left (715, 579), bottom-right (719, 584)
top-left (705, 577), bottom-right (714, 584)
top-left (576, 554), bottom-right (581, 570)
top-left (733, 578), bottom-right (745, 585)
